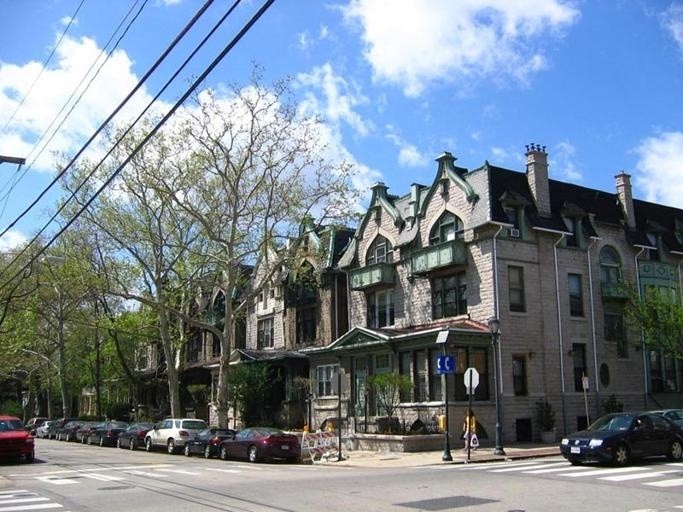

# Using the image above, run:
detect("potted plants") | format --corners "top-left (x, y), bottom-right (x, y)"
top-left (535, 400), bottom-right (558, 443)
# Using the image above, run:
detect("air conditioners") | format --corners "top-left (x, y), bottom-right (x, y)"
top-left (510, 227), bottom-right (521, 238)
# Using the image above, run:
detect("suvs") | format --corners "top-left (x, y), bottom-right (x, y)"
top-left (144, 418), bottom-right (208, 454)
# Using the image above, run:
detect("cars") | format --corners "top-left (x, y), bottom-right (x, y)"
top-left (183, 429), bottom-right (235, 459)
top-left (218, 426), bottom-right (300, 463)
top-left (0, 416), bottom-right (35, 462)
top-left (117, 422), bottom-right (153, 450)
top-left (560, 409), bottom-right (683, 465)
top-left (25, 417), bottom-right (129, 448)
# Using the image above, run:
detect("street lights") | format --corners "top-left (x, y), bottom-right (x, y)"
top-left (488, 317), bottom-right (505, 455)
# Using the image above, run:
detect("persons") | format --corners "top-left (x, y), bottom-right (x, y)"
top-left (326, 415), bottom-right (335, 432)
top-left (463, 408), bottom-right (477, 451)
top-left (303, 424), bottom-right (309, 432)
